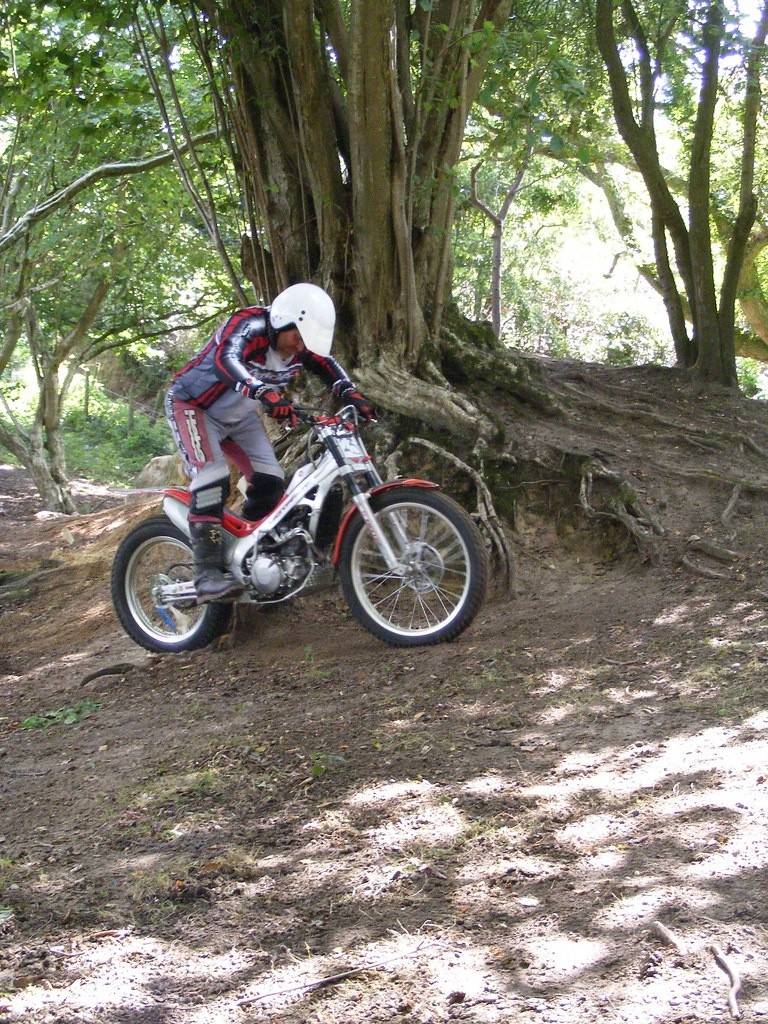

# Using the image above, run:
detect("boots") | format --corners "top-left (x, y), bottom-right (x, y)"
top-left (188, 522), bottom-right (245, 604)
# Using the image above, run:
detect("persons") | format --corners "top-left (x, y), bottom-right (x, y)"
top-left (164, 283), bottom-right (383, 611)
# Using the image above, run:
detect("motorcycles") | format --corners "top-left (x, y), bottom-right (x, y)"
top-left (108, 397), bottom-right (488, 655)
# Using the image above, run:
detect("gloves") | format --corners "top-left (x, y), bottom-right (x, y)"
top-left (256, 388), bottom-right (297, 427)
top-left (331, 379), bottom-right (382, 423)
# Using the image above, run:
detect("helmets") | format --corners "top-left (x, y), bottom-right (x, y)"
top-left (269, 282), bottom-right (337, 358)
